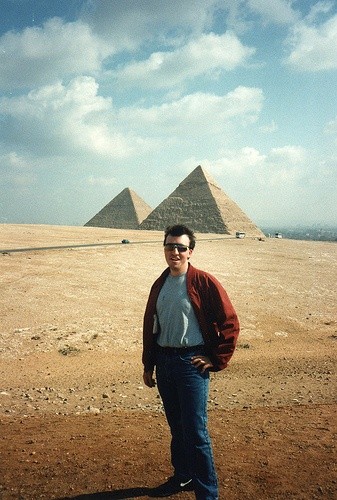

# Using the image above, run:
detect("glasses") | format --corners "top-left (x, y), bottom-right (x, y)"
top-left (163, 243), bottom-right (192, 252)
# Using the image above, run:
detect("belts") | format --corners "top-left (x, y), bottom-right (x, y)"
top-left (156, 346), bottom-right (192, 356)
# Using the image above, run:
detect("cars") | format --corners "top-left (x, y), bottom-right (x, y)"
top-left (122, 239), bottom-right (129, 244)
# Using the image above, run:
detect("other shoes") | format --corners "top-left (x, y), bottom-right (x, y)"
top-left (149, 477), bottom-right (194, 498)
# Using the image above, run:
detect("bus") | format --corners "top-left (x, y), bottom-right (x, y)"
top-left (275, 233), bottom-right (282, 238)
top-left (236, 232), bottom-right (246, 238)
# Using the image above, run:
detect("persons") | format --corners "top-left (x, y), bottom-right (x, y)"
top-left (142, 223), bottom-right (240, 500)
